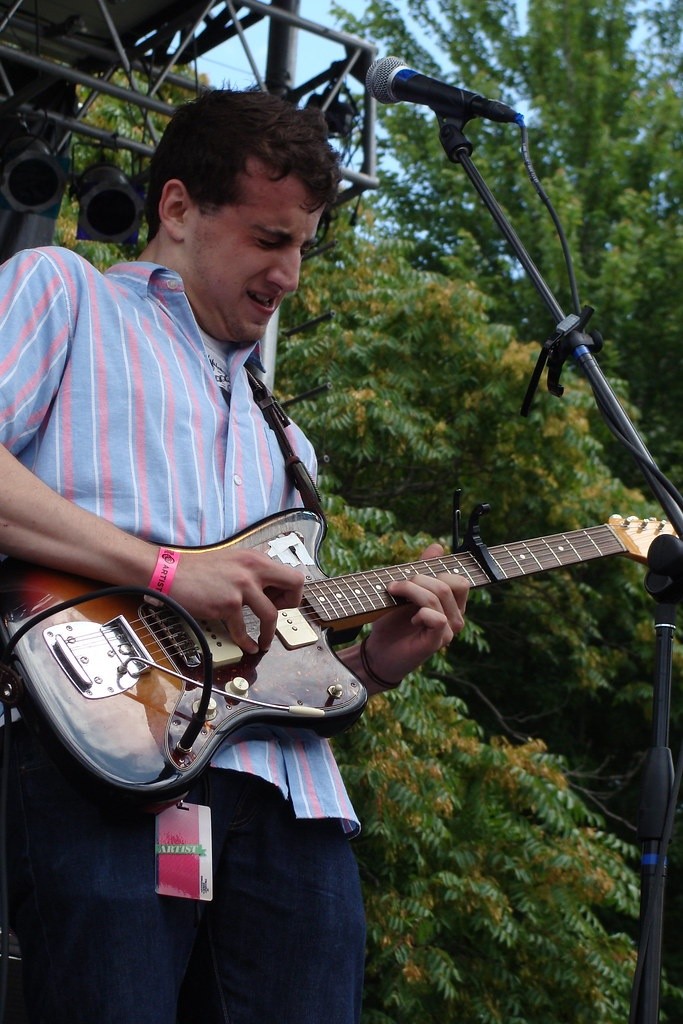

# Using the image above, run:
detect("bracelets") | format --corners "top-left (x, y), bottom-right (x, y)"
top-left (360, 631), bottom-right (402, 689)
top-left (144, 546), bottom-right (181, 607)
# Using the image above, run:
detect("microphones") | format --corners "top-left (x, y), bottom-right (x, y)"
top-left (366, 55), bottom-right (521, 123)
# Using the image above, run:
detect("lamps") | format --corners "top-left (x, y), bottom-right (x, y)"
top-left (0, 105), bottom-right (144, 244)
top-left (303, 81), bottom-right (355, 135)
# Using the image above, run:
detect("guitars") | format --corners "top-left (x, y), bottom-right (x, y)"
top-left (0, 507), bottom-right (680, 817)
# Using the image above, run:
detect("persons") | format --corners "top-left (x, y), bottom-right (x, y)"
top-left (0, 89), bottom-right (471, 1024)
